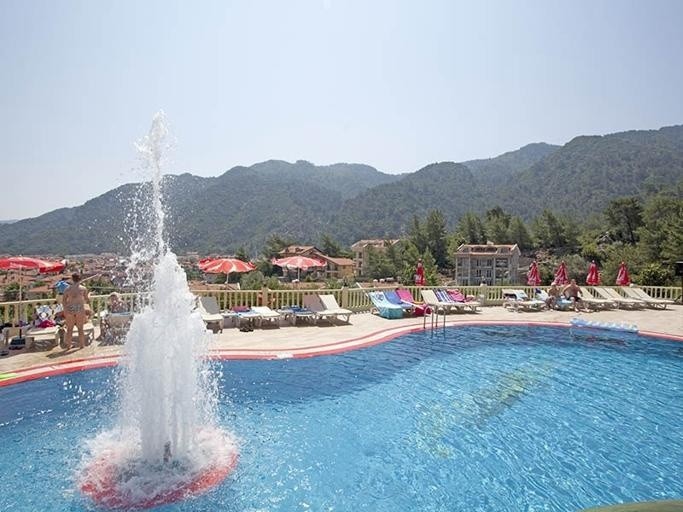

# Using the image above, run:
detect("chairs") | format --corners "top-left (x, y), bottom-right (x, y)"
top-left (501, 282), bottom-right (675, 312)
top-left (367, 287), bottom-right (482, 320)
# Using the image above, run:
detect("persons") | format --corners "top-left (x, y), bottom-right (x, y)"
top-left (257, 283), bottom-right (275, 306)
top-left (61, 272), bottom-right (91, 349)
top-left (94, 291), bottom-right (129, 341)
top-left (562, 278), bottom-right (587, 312)
top-left (544, 281), bottom-right (560, 311)
top-left (48, 273), bottom-right (67, 304)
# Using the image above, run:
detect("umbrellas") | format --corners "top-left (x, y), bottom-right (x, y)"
top-left (269, 253), bottom-right (329, 284)
top-left (526, 260), bottom-right (541, 286)
top-left (550, 260), bottom-right (568, 286)
top-left (615, 260), bottom-right (630, 286)
top-left (192, 257), bottom-right (256, 284)
top-left (413, 257), bottom-right (426, 286)
top-left (585, 258), bottom-right (600, 286)
top-left (0, 253), bottom-right (64, 303)
